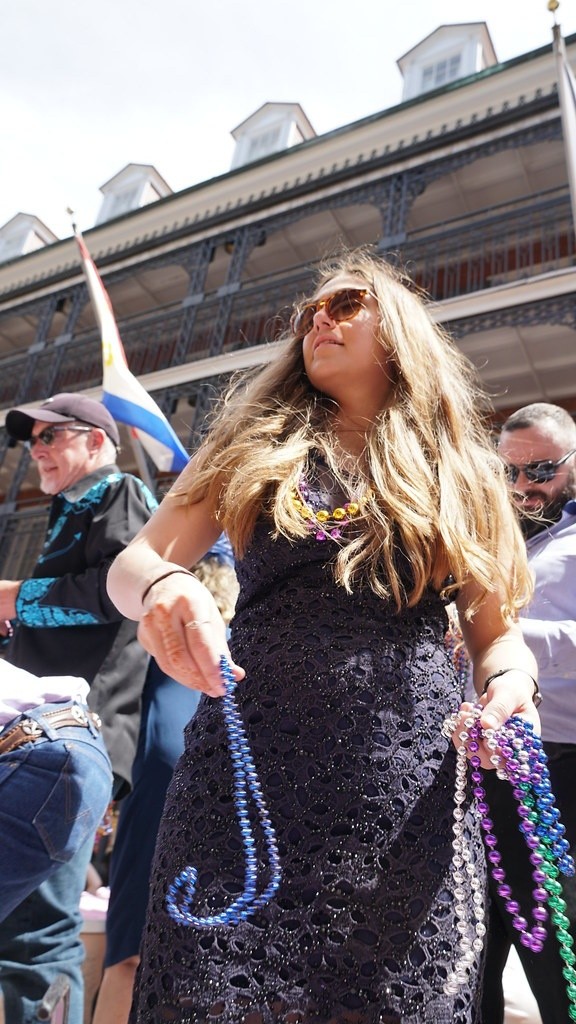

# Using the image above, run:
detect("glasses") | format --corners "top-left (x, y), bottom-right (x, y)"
top-left (291, 288), bottom-right (375, 337)
top-left (25, 424), bottom-right (94, 452)
top-left (494, 448), bottom-right (576, 485)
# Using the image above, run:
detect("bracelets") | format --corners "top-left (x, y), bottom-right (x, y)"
top-left (481, 668), bottom-right (543, 710)
top-left (142, 570), bottom-right (201, 602)
top-left (4, 619), bottom-right (12, 637)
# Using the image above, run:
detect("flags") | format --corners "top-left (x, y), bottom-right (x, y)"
top-left (70, 223), bottom-right (192, 472)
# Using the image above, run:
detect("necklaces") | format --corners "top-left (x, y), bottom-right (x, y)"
top-left (290, 472), bottom-right (374, 542)
top-left (447, 636), bottom-right (471, 693)
top-left (164, 654), bottom-right (281, 928)
top-left (442, 702), bottom-right (576, 1023)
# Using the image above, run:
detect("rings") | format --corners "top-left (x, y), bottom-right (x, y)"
top-left (185, 619), bottom-right (210, 628)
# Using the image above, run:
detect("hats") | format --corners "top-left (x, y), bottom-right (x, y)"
top-left (6, 392), bottom-right (121, 455)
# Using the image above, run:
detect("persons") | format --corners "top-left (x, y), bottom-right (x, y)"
top-left (444, 403), bottom-right (576, 1024)
top-left (0, 393), bottom-right (239, 1024)
top-left (106, 250), bottom-right (543, 1024)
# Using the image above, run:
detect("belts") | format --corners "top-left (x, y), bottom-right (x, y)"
top-left (0, 705), bottom-right (100, 756)
